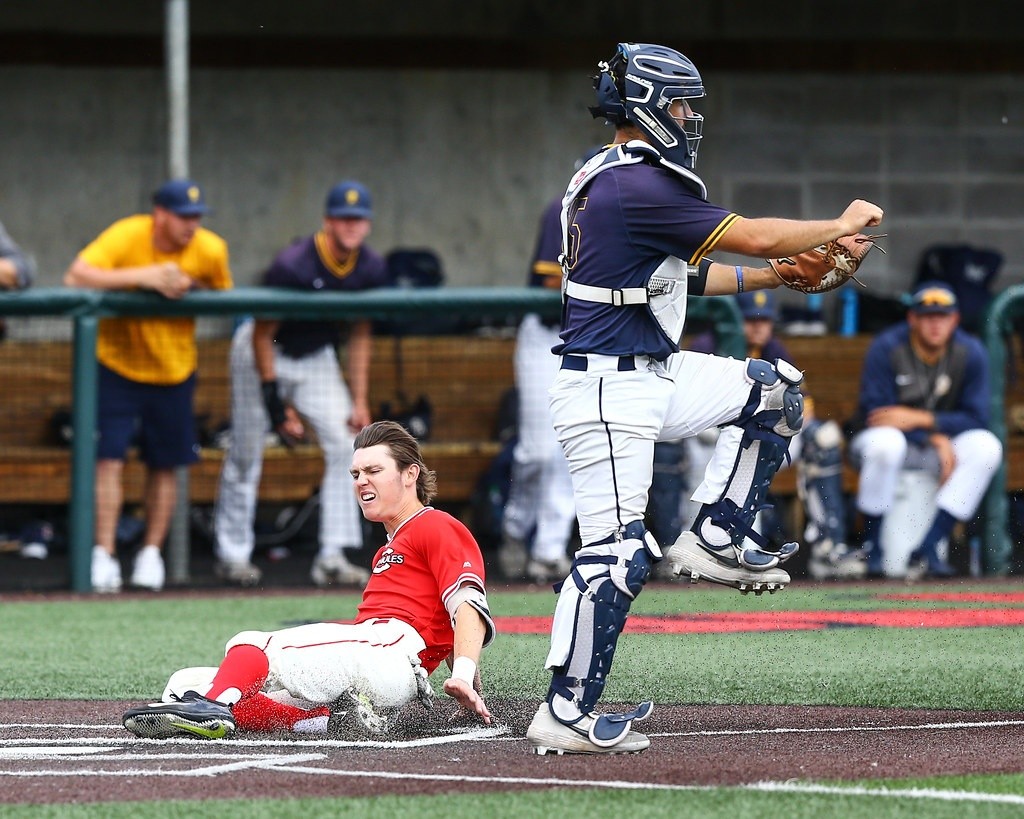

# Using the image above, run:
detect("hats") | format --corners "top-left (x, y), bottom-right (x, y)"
top-left (325, 182), bottom-right (372, 219)
top-left (907, 281), bottom-right (959, 314)
top-left (151, 178), bottom-right (211, 215)
top-left (737, 290), bottom-right (779, 320)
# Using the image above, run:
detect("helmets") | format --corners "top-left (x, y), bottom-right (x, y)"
top-left (588, 42), bottom-right (706, 170)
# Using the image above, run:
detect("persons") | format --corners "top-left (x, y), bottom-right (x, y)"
top-left (123, 420), bottom-right (498, 742)
top-left (527, 41), bottom-right (886, 759)
top-left (0, 181), bottom-right (1005, 590)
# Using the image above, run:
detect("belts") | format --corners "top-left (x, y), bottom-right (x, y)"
top-left (560, 354), bottom-right (636, 371)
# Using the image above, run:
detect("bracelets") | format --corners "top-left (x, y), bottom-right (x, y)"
top-left (735, 265), bottom-right (743, 292)
top-left (450, 658), bottom-right (478, 688)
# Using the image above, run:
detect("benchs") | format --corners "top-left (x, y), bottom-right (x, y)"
top-left (0, 336), bottom-right (1024, 508)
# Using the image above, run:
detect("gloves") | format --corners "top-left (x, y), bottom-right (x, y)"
top-left (408, 654), bottom-right (435, 710)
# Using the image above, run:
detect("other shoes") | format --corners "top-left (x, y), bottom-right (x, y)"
top-left (907, 552), bottom-right (958, 579)
top-left (132, 545), bottom-right (165, 590)
top-left (497, 533), bottom-right (526, 577)
top-left (90, 546), bottom-right (123, 594)
top-left (213, 558), bottom-right (262, 586)
top-left (866, 555), bottom-right (884, 577)
top-left (807, 553), bottom-right (866, 580)
top-left (526, 556), bottom-right (573, 580)
top-left (309, 557), bottom-right (369, 585)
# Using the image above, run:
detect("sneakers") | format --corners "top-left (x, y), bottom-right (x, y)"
top-left (121, 688), bottom-right (236, 740)
top-left (328, 686), bottom-right (393, 743)
top-left (526, 701), bottom-right (650, 755)
top-left (667, 531), bottom-right (791, 596)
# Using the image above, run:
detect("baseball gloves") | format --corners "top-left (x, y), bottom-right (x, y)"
top-left (768, 232), bottom-right (875, 294)
